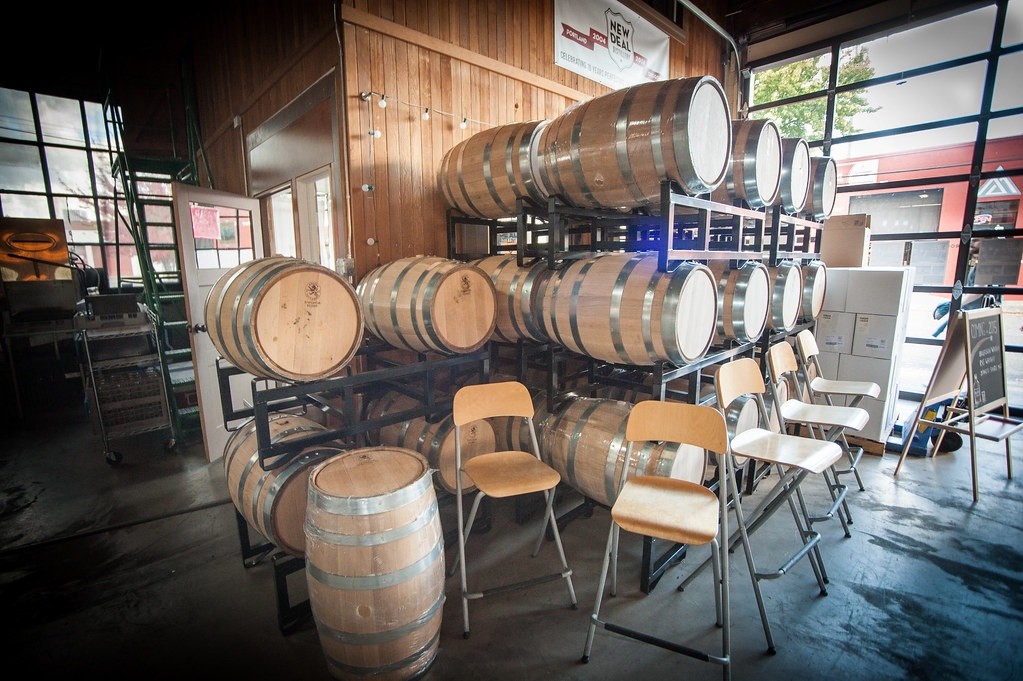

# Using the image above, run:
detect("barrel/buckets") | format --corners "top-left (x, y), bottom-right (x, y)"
top-left (204, 255), bottom-right (365, 380)
top-left (361, 382), bottom-right (498, 496)
top-left (305, 446), bottom-right (447, 681)
top-left (538, 75), bottom-right (733, 210)
top-left (356, 254), bottom-right (498, 357)
top-left (222, 412), bottom-right (349, 554)
top-left (440, 120), bottom-right (839, 509)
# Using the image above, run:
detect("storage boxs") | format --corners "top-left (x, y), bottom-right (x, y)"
top-left (821, 268), bottom-right (848, 312)
top-left (843, 395), bottom-right (894, 441)
top-left (845, 267), bottom-right (910, 316)
top-left (838, 353), bottom-right (897, 402)
top-left (816, 311), bottom-right (856, 354)
top-left (851, 314), bottom-right (904, 359)
top-left (810, 392), bottom-right (847, 431)
top-left (817, 352), bottom-right (840, 380)
top-left (821, 213), bottom-right (871, 267)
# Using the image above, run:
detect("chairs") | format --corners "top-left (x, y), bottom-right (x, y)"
top-left (580, 401), bottom-right (731, 681)
top-left (763, 328), bottom-right (881, 525)
top-left (445, 381), bottom-right (579, 639)
top-left (729, 341), bottom-right (870, 584)
top-left (676, 359), bottom-right (843, 656)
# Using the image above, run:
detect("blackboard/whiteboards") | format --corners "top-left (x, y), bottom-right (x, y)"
top-left (960, 306), bottom-right (1008, 416)
